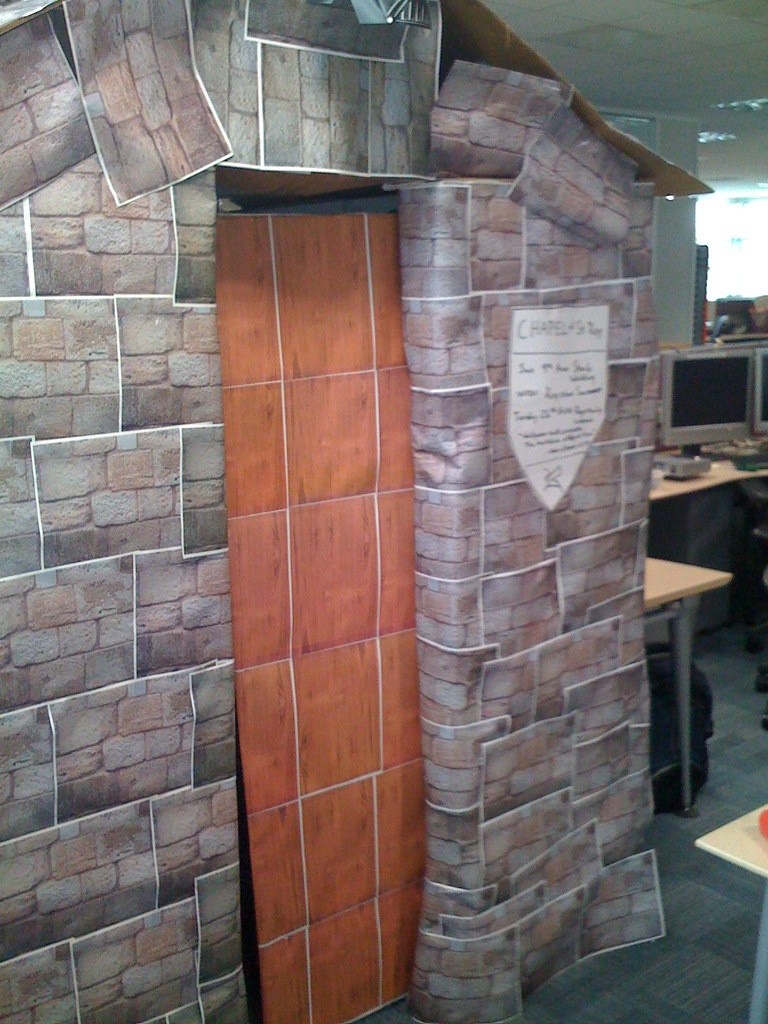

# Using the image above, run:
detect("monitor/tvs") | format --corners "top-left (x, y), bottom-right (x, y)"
top-left (662, 348), bottom-right (755, 463)
top-left (753, 348), bottom-right (768, 434)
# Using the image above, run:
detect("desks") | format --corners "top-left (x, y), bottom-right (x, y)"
top-left (695, 800), bottom-right (768, 1024)
top-left (643, 557), bottom-right (734, 816)
top-left (649, 453), bottom-right (761, 570)
